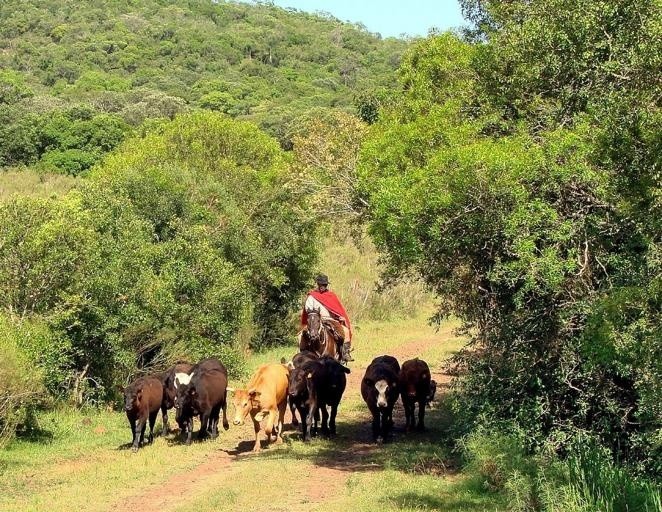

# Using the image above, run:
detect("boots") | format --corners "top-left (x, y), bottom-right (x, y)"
top-left (343, 343), bottom-right (354, 361)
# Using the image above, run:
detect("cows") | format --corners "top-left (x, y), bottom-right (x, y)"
top-left (401, 357), bottom-right (431, 434)
top-left (148, 360), bottom-right (193, 437)
top-left (361, 355), bottom-right (401, 443)
top-left (115, 375), bottom-right (163, 453)
top-left (226, 361), bottom-right (291, 453)
top-left (318, 354), bottom-right (351, 438)
top-left (285, 360), bottom-right (320, 445)
top-left (172, 356), bottom-right (228, 439)
top-left (280, 350), bottom-right (319, 371)
top-left (426, 380), bottom-right (438, 411)
top-left (173, 369), bottom-right (228, 446)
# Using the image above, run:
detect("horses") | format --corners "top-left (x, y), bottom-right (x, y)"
top-left (299, 306), bottom-right (342, 362)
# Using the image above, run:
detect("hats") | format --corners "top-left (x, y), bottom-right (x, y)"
top-left (316, 275), bottom-right (331, 285)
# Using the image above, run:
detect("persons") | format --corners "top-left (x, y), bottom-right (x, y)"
top-left (297, 274), bottom-right (354, 361)
